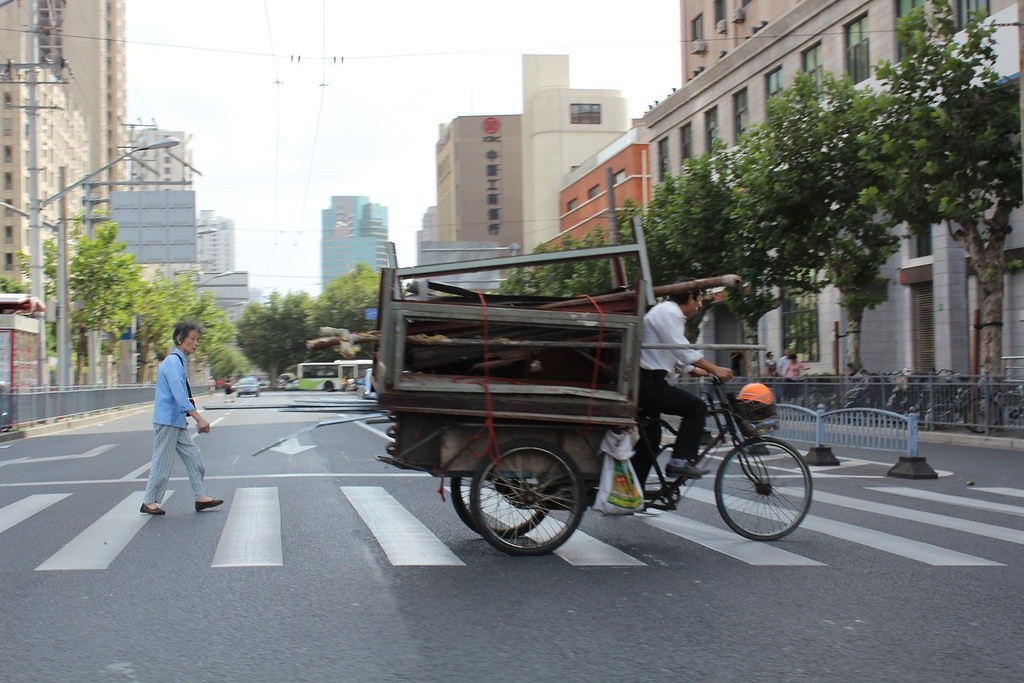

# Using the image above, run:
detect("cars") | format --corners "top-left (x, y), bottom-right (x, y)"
top-left (235, 378), bottom-right (260, 398)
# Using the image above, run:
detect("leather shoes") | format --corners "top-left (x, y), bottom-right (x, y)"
top-left (664, 461), bottom-right (709, 478)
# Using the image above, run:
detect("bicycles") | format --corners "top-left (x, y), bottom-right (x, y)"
top-left (908, 367), bottom-right (1002, 433)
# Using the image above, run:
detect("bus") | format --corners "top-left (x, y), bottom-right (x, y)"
top-left (297, 359), bottom-right (373, 392)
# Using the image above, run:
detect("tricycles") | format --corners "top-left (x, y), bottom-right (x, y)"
top-left (377, 371), bottom-right (817, 556)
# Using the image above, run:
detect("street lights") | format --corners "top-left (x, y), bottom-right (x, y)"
top-left (28, 136), bottom-right (180, 425)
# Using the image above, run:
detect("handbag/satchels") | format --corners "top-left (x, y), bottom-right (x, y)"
top-left (186, 397), bottom-right (196, 417)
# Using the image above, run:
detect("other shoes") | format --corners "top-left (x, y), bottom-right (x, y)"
top-left (194, 497), bottom-right (226, 513)
top-left (138, 502), bottom-right (166, 517)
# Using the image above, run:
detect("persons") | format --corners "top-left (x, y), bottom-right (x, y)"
top-left (778, 348), bottom-right (792, 382)
top-left (630, 284), bottom-right (736, 512)
top-left (764, 351), bottom-right (780, 403)
top-left (781, 354), bottom-right (808, 401)
top-left (208, 375), bottom-right (216, 398)
top-left (139, 321), bottom-right (224, 516)
top-left (223, 375), bottom-right (236, 403)
top-left (729, 349), bottom-right (744, 377)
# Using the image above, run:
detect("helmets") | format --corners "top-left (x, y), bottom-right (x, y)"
top-left (736, 381), bottom-right (774, 407)
